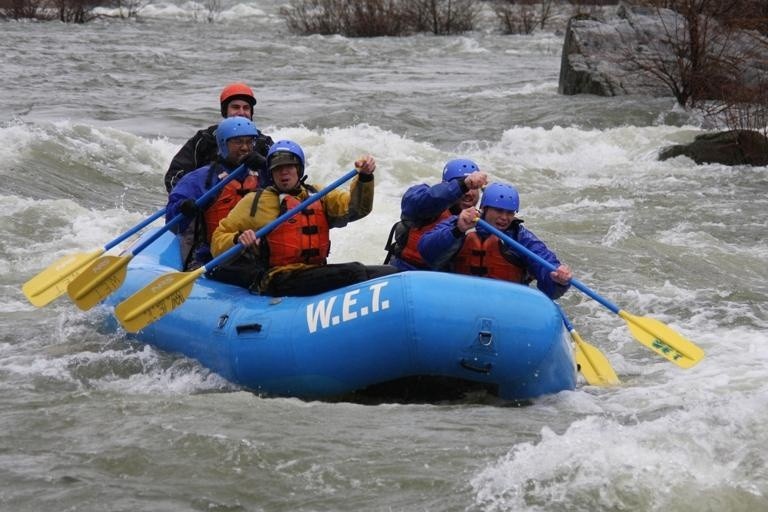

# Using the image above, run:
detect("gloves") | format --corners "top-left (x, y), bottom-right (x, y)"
top-left (179, 198), bottom-right (198, 218)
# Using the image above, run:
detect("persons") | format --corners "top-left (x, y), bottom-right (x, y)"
top-left (165, 116), bottom-right (268, 288)
top-left (418, 181), bottom-right (571, 300)
top-left (211, 141), bottom-right (397, 298)
top-left (384, 159), bottom-right (488, 273)
top-left (165, 83), bottom-right (275, 192)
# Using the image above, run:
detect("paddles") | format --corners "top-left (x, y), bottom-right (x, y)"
top-left (20, 206), bottom-right (167, 309)
top-left (471, 209), bottom-right (705, 370)
top-left (480, 179), bottom-right (623, 387)
top-left (66, 162), bottom-right (247, 312)
top-left (113, 154), bottom-right (370, 334)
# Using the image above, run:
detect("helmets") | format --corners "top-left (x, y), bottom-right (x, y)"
top-left (220, 83), bottom-right (257, 120)
top-left (216, 116), bottom-right (258, 160)
top-left (264, 139), bottom-right (305, 167)
top-left (480, 181), bottom-right (519, 213)
top-left (442, 158), bottom-right (480, 185)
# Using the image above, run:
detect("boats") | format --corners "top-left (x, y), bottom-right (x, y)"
top-left (97, 225), bottom-right (577, 405)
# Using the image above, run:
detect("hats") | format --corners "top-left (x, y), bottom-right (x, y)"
top-left (268, 152), bottom-right (301, 171)
